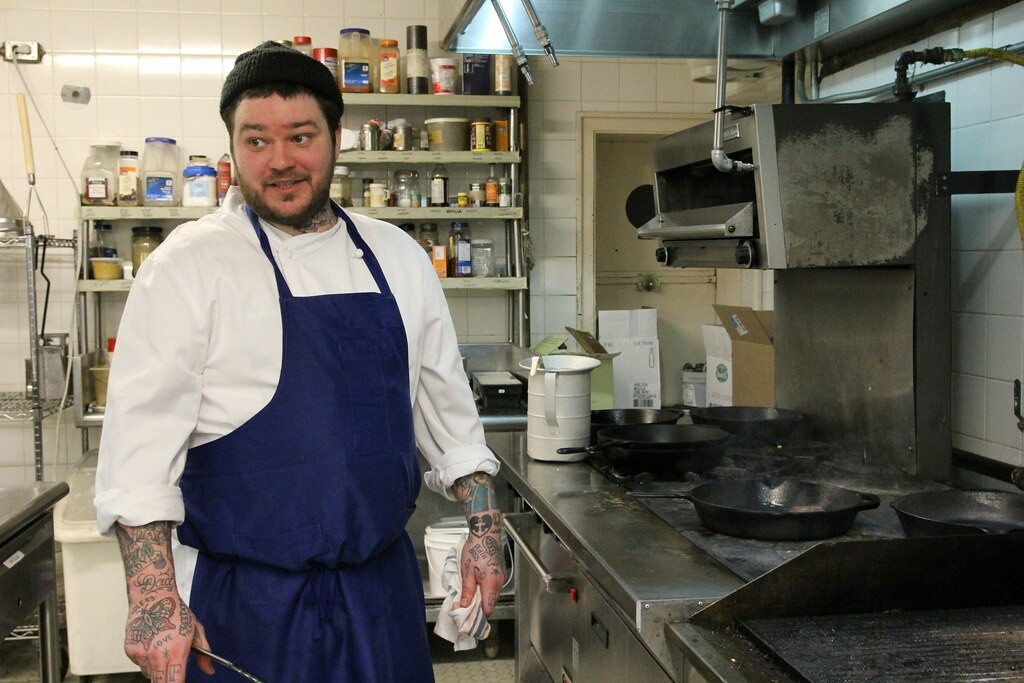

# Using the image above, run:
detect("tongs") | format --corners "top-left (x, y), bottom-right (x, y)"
top-left (191, 645), bottom-right (263, 683)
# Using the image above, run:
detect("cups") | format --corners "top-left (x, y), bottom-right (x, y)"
top-left (369, 184), bottom-right (390, 207)
top-left (431, 58), bottom-right (458, 94)
top-left (90, 258), bottom-right (124, 279)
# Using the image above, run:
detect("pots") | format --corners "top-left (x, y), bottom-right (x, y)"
top-left (556, 424), bottom-right (735, 473)
top-left (626, 479), bottom-right (880, 541)
top-left (673, 404), bottom-right (815, 436)
top-left (592, 409), bottom-right (685, 427)
top-left (890, 490), bottom-right (1024, 538)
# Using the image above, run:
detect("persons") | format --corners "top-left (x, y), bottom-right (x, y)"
top-left (94, 41), bottom-right (506, 683)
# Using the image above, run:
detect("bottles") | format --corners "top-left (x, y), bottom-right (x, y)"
top-left (488, 54), bottom-right (512, 95)
top-left (458, 192), bottom-right (468, 207)
top-left (183, 156), bottom-right (217, 206)
top-left (360, 124), bottom-right (413, 151)
top-left (89, 224), bottom-right (163, 278)
top-left (499, 177), bottom-right (512, 206)
top-left (362, 178), bottom-right (374, 207)
top-left (394, 169), bottom-right (421, 207)
top-left (485, 163), bottom-right (499, 207)
top-left (468, 182), bottom-right (485, 207)
top-left (398, 223), bottom-right (416, 241)
top-left (430, 163), bottom-right (450, 207)
top-left (419, 223), bottom-right (440, 264)
top-left (273, 25), bottom-right (428, 94)
top-left (471, 239), bottom-right (495, 277)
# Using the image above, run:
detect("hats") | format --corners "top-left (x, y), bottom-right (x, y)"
top-left (220, 41), bottom-right (344, 119)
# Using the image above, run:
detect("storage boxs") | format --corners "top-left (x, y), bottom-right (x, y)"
top-left (711, 303), bottom-right (775, 407)
top-left (51, 447), bottom-right (200, 677)
top-left (534, 326), bottom-right (622, 408)
top-left (702, 322), bottom-right (731, 406)
top-left (472, 371), bottom-right (525, 409)
top-left (597, 305), bottom-right (659, 409)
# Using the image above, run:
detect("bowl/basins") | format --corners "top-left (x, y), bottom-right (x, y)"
top-left (424, 118), bottom-right (470, 151)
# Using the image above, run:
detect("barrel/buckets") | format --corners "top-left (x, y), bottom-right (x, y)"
top-left (423, 520), bottom-right (508, 595)
top-left (681, 365), bottom-right (706, 408)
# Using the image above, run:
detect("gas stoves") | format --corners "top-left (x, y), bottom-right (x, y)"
top-left (586, 428), bottom-right (843, 481)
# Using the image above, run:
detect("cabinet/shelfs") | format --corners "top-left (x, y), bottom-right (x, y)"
top-left (71, 92), bottom-right (530, 657)
top-left (1, 218), bottom-right (92, 641)
